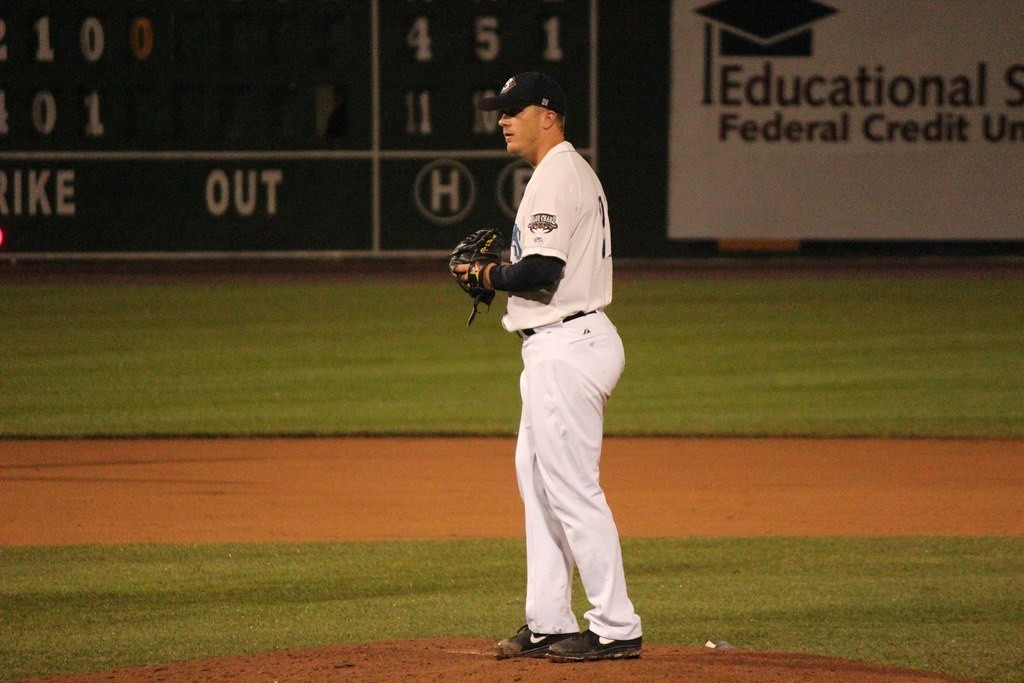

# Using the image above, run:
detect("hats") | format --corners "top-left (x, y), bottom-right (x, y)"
top-left (478, 70), bottom-right (567, 113)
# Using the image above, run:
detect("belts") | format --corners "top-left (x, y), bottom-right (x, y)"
top-left (517, 309), bottom-right (596, 339)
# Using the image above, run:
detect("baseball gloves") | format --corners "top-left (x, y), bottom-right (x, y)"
top-left (448, 227), bottom-right (504, 328)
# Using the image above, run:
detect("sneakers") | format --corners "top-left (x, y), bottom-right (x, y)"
top-left (495, 627), bottom-right (646, 663)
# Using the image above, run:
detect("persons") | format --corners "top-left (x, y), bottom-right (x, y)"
top-left (449, 71), bottom-right (642, 661)
